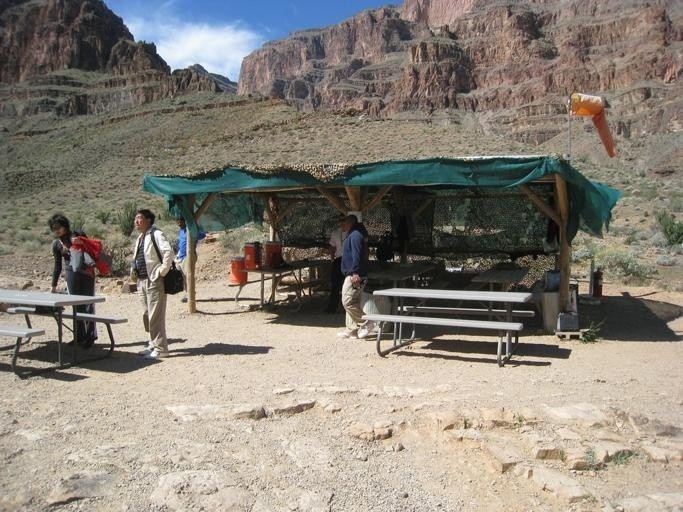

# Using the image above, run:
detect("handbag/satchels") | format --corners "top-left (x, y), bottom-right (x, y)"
top-left (150, 226), bottom-right (187, 295)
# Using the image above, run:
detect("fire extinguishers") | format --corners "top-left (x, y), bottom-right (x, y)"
top-left (593, 267), bottom-right (603, 297)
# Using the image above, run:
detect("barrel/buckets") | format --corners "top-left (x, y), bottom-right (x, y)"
top-left (265, 242), bottom-right (282, 266)
top-left (245, 243), bottom-right (260, 270)
top-left (231, 256), bottom-right (247, 285)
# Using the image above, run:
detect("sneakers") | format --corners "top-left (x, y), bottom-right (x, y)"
top-left (137, 348), bottom-right (167, 360)
top-left (338, 321), bottom-right (376, 340)
top-left (68, 339), bottom-right (95, 350)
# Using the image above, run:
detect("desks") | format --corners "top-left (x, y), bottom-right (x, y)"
top-left (241, 258), bottom-right (329, 308)
top-left (375, 262), bottom-right (437, 309)
top-left (0, 288), bottom-right (105, 370)
top-left (473, 265), bottom-right (530, 316)
top-left (372, 286), bottom-right (534, 356)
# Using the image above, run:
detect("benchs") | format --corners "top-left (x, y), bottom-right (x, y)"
top-left (521, 280), bottom-right (546, 324)
top-left (276, 276), bottom-right (327, 312)
top-left (398, 304), bottom-right (537, 344)
top-left (0, 322), bottom-right (44, 378)
top-left (227, 271), bottom-right (294, 307)
top-left (456, 283), bottom-right (486, 308)
top-left (413, 279), bottom-right (451, 317)
top-left (360, 313), bottom-right (524, 367)
top-left (7, 305), bottom-right (130, 357)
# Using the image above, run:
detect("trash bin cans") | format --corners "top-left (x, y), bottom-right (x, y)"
top-left (360, 282), bottom-right (395, 333)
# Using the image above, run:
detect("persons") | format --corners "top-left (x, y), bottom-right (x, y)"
top-left (337, 214), bottom-right (378, 340)
top-left (173, 216), bottom-right (206, 303)
top-left (131, 209), bottom-right (175, 359)
top-left (325, 214), bottom-right (348, 313)
top-left (48, 213), bottom-right (98, 349)
